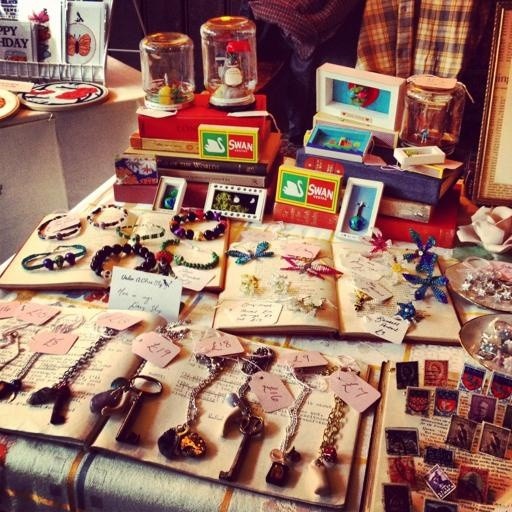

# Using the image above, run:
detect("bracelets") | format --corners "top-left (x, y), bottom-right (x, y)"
top-left (218, 347), bottom-right (277, 483)
top-left (0, 311), bottom-right (86, 402)
top-left (159, 239), bottom-right (220, 270)
top-left (313, 365), bottom-right (362, 497)
top-left (37, 215), bottom-right (82, 241)
top-left (22, 244), bottom-right (87, 270)
top-left (156, 352), bottom-right (224, 460)
top-left (169, 207), bottom-right (228, 242)
top-left (90, 243), bottom-right (156, 281)
top-left (25, 326), bottom-right (119, 427)
top-left (86, 204), bottom-right (130, 229)
top-left (265, 364), bottom-right (312, 489)
top-left (116, 224), bottom-right (166, 241)
top-left (0, 323), bottom-right (32, 371)
top-left (89, 318), bottom-right (191, 444)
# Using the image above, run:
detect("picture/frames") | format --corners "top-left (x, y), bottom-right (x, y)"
top-left (468, 1), bottom-right (512, 210)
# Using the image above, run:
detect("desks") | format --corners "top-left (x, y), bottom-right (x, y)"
top-left (1, 55), bottom-right (147, 262)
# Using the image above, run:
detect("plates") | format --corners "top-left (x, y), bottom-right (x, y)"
top-left (459, 312), bottom-right (511, 381)
top-left (443, 258), bottom-right (511, 313)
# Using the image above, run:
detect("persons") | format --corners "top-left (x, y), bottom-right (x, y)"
top-left (455, 423), bottom-right (468, 446)
top-left (487, 431), bottom-right (502, 454)
top-left (472, 400), bottom-right (492, 423)
